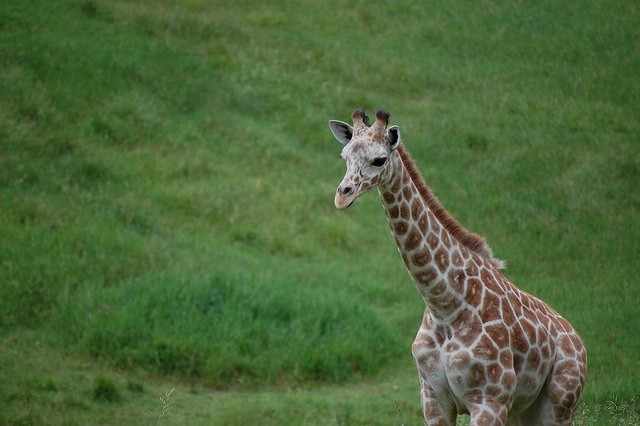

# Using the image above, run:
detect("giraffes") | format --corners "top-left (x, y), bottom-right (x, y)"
top-left (329, 108), bottom-right (588, 426)
